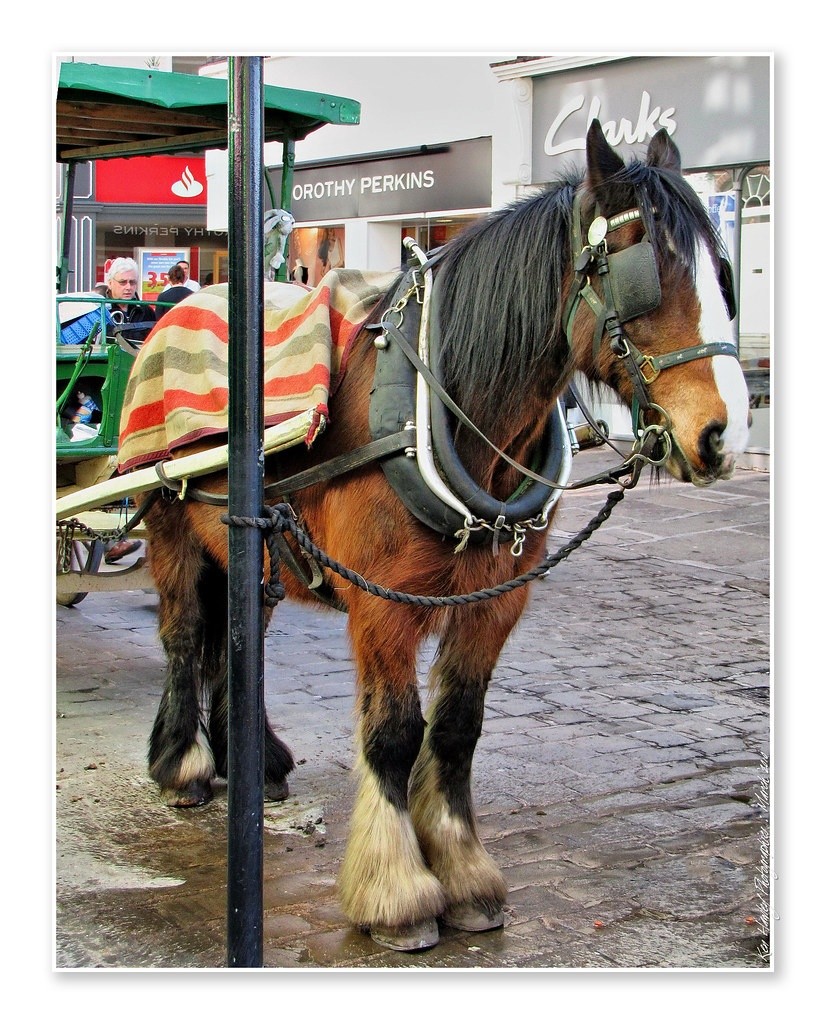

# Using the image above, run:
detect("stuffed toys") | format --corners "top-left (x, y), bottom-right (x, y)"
top-left (263, 209), bottom-right (295, 280)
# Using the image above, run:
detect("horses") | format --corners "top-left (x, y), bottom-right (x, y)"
top-left (117, 120), bottom-right (749, 950)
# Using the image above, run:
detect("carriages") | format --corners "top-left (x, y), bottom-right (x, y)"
top-left (59, 57), bottom-right (759, 953)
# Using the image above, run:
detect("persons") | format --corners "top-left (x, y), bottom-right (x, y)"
top-left (155, 265), bottom-right (194, 322)
top-left (319, 227), bottom-right (343, 277)
top-left (92, 257), bottom-right (156, 564)
top-left (290, 259), bottom-right (308, 285)
top-left (163, 261), bottom-right (201, 292)
